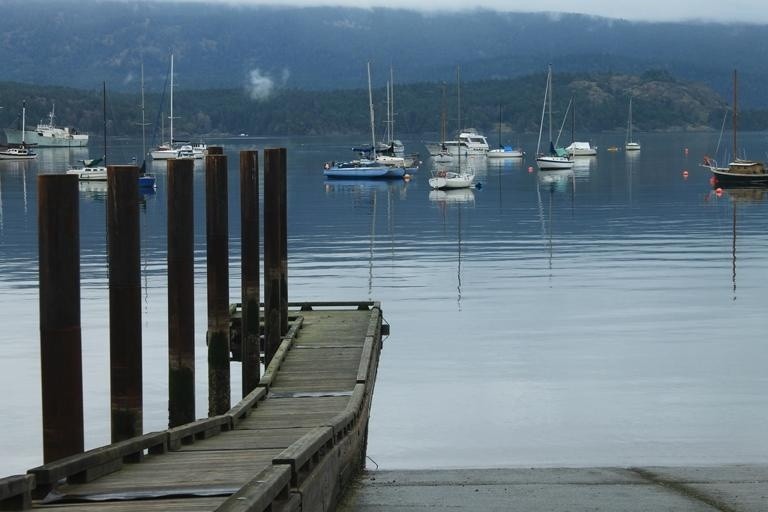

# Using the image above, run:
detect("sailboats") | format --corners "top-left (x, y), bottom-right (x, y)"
top-left (623, 98), bottom-right (640, 150)
top-left (428, 61), bottom-right (476, 189)
top-left (65, 79), bottom-right (129, 181)
top-left (146, 52), bottom-right (209, 160)
top-left (130, 62), bottom-right (155, 188)
top-left (323, 63), bottom-right (422, 184)
top-left (534, 63), bottom-right (574, 169)
top-left (0, 100), bottom-right (38, 159)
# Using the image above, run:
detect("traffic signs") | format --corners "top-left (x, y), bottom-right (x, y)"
top-left (421, 132), bottom-right (489, 156)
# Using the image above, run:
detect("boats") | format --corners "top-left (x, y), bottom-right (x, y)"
top-left (564, 142), bottom-right (597, 155)
top-left (709, 70), bottom-right (766, 187)
top-left (485, 145), bottom-right (525, 158)
top-left (0, 102), bottom-right (88, 146)
